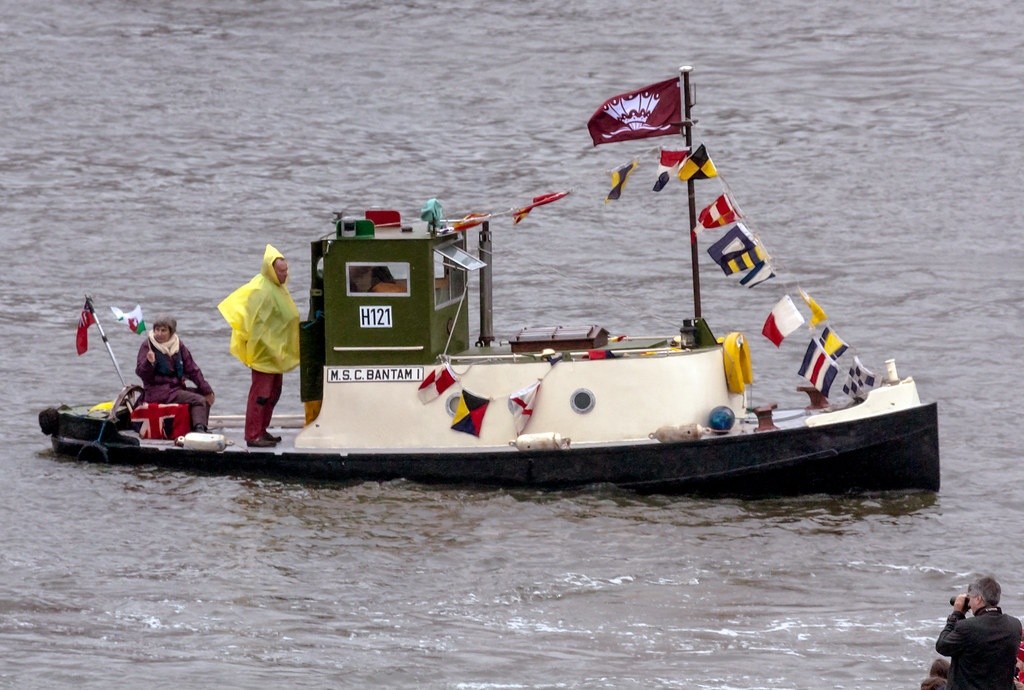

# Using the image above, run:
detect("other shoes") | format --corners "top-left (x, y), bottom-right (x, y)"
top-left (263, 432), bottom-right (282, 442)
top-left (247, 438), bottom-right (276, 447)
top-left (194, 426), bottom-right (209, 433)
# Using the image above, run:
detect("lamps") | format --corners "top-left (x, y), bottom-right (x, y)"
top-left (681, 319), bottom-right (699, 351)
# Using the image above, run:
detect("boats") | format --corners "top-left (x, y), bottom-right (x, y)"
top-left (36, 63), bottom-right (941, 498)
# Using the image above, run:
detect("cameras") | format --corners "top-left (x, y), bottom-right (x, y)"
top-left (949, 596), bottom-right (971, 611)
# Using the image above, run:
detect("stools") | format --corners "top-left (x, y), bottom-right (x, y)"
top-left (130, 402), bottom-right (192, 441)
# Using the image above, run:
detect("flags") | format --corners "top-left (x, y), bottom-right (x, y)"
top-left (111, 307), bottom-right (146, 335)
top-left (76, 297), bottom-right (96, 355)
top-left (419, 144), bottom-right (882, 439)
top-left (586, 76), bottom-right (682, 147)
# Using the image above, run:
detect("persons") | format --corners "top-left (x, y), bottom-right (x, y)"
top-left (921, 630), bottom-right (1024, 690)
top-left (935, 579), bottom-right (1022, 690)
top-left (349, 265), bottom-right (396, 293)
top-left (135, 315), bottom-right (215, 435)
top-left (218, 244), bottom-right (300, 448)
